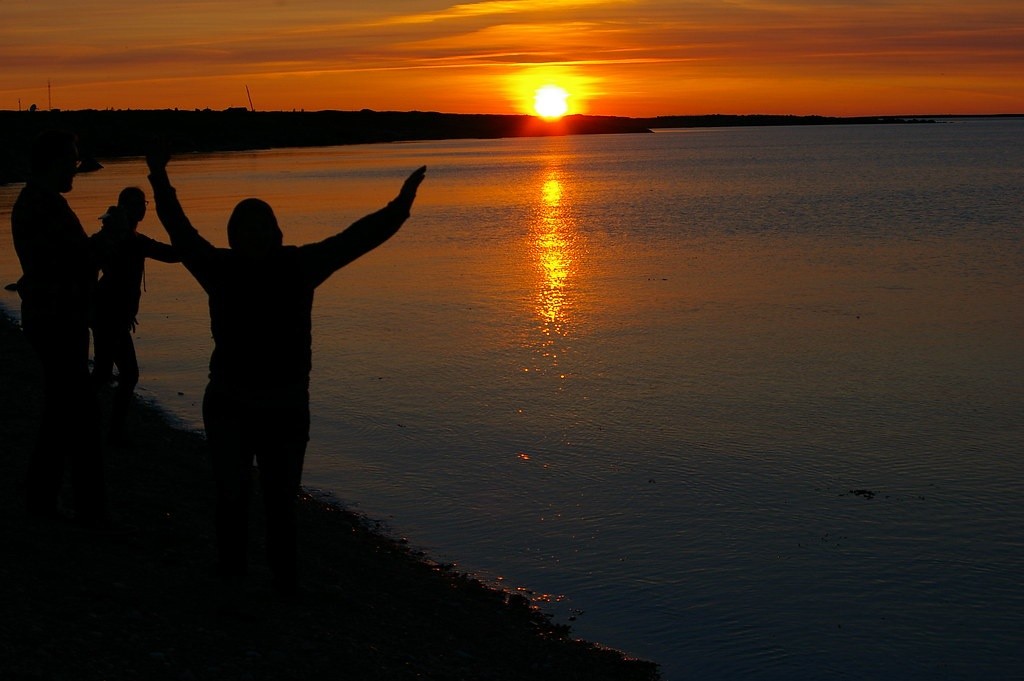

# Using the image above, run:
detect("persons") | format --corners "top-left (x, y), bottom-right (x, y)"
top-left (10, 132), bottom-right (190, 397)
top-left (147, 140), bottom-right (426, 484)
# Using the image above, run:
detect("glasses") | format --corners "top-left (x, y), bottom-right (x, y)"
top-left (143, 201), bottom-right (149, 206)
top-left (65, 159), bottom-right (82, 168)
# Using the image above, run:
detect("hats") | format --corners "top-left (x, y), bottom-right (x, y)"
top-left (98, 206), bottom-right (116, 219)
